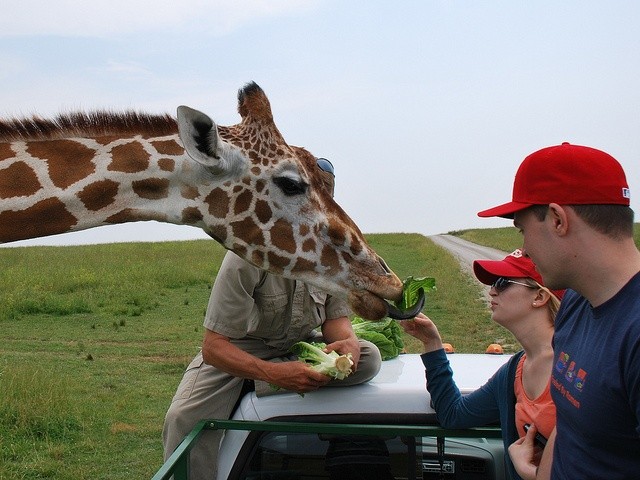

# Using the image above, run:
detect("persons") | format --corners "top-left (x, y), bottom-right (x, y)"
top-left (161, 157), bottom-right (382, 480)
top-left (477, 142), bottom-right (640, 480)
top-left (398, 248), bottom-right (569, 480)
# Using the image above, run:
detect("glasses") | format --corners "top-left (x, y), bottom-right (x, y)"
top-left (490, 277), bottom-right (536, 293)
top-left (315, 158), bottom-right (335, 175)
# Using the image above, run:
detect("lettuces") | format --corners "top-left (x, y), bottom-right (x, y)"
top-left (394, 276), bottom-right (439, 317)
top-left (350, 316), bottom-right (405, 361)
top-left (265, 340), bottom-right (355, 399)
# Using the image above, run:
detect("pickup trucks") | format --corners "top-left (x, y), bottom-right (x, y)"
top-left (150, 343), bottom-right (527, 480)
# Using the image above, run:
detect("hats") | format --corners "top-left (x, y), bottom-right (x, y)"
top-left (473, 246), bottom-right (568, 300)
top-left (476, 142), bottom-right (630, 217)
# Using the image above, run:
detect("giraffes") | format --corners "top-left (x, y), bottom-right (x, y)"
top-left (0, 79), bottom-right (426, 325)
